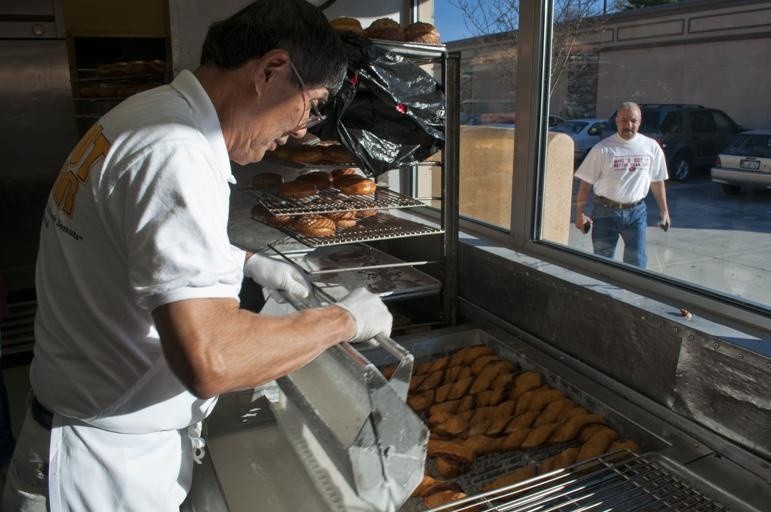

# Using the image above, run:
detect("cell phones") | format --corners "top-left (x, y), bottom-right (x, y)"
top-left (659, 216), bottom-right (668, 232)
top-left (585, 221), bottom-right (590, 233)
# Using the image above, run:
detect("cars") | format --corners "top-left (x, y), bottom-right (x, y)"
top-left (458, 102), bottom-right (747, 187)
top-left (709, 126), bottom-right (771, 196)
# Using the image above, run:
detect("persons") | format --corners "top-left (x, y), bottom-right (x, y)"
top-left (574, 102), bottom-right (670, 269)
top-left (0, 0), bottom-right (394, 512)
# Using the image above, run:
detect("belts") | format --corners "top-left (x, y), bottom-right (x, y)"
top-left (594, 196), bottom-right (643, 209)
top-left (30, 394), bottom-right (52, 431)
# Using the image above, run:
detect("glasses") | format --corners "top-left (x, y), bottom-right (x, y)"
top-left (290, 60), bottom-right (327, 128)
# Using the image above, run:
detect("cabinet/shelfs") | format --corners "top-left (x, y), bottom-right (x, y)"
top-left (69, 55), bottom-right (171, 137)
top-left (235, 36), bottom-right (461, 326)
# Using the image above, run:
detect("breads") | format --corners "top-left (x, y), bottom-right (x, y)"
top-left (330, 18), bottom-right (440, 43)
top-left (269, 132), bottom-right (378, 237)
top-left (379, 346), bottom-right (640, 512)
top-left (96, 59), bottom-right (165, 97)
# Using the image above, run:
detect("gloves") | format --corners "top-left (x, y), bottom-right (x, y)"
top-left (334, 286), bottom-right (394, 351)
top-left (243, 252), bottom-right (312, 303)
